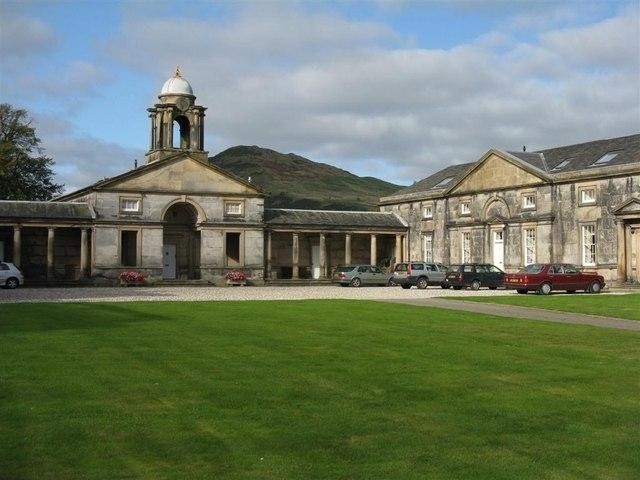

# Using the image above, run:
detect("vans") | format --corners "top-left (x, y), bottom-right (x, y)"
top-left (392, 262), bottom-right (449, 289)
top-left (445, 264), bottom-right (507, 291)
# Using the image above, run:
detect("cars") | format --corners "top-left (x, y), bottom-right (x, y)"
top-left (504, 261), bottom-right (606, 296)
top-left (0, 262), bottom-right (25, 290)
top-left (332, 264), bottom-right (398, 287)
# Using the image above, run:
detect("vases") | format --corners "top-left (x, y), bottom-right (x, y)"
top-left (121, 278), bottom-right (142, 288)
top-left (226, 279), bottom-right (245, 287)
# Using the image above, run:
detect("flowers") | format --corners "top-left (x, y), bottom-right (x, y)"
top-left (224, 269), bottom-right (245, 281)
top-left (119, 272), bottom-right (143, 282)
